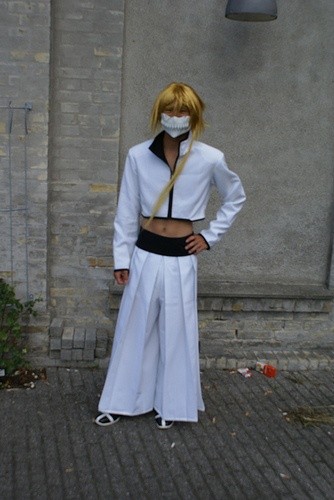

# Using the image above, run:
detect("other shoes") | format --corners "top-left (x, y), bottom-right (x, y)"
top-left (95, 414), bottom-right (123, 426)
top-left (155, 411), bottom-right (175, 429)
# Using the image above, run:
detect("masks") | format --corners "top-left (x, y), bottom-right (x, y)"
top-left (160, 113), bottom-right (191, 138)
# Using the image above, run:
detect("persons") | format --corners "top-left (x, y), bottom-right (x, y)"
top-left (93, 82), bottom-right (246, 429)
